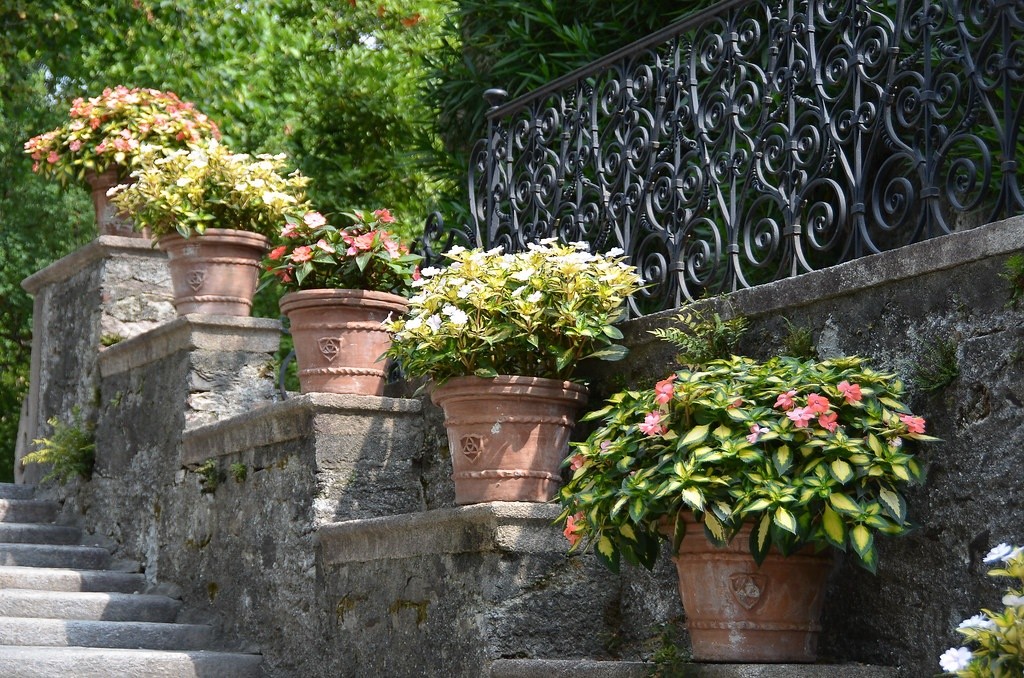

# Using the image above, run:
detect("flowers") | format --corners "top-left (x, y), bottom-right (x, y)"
top-left (379, 237), bottom-right (645, 381)
top-left (554, 353), bottom-right (944, 574)
top-left (22, 84), bottom-right (221, 186)
top-left (104, 135), bottom-right (313, 247)
top-left (261, 206), bottom-right (420, 301)
top-left (937, 543), bottom-right (1024, 678)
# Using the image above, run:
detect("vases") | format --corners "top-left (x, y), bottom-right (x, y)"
top-left (157, 228), bottom-right (272, 319)
top-left (85, 168), bottom-right (156, 240)
top-left (432, 375), bottom-right (590, 503)
top-left (662, 510), bottom-right (846, 662)
top-left (278, 288), bottom-right (411, 395)
